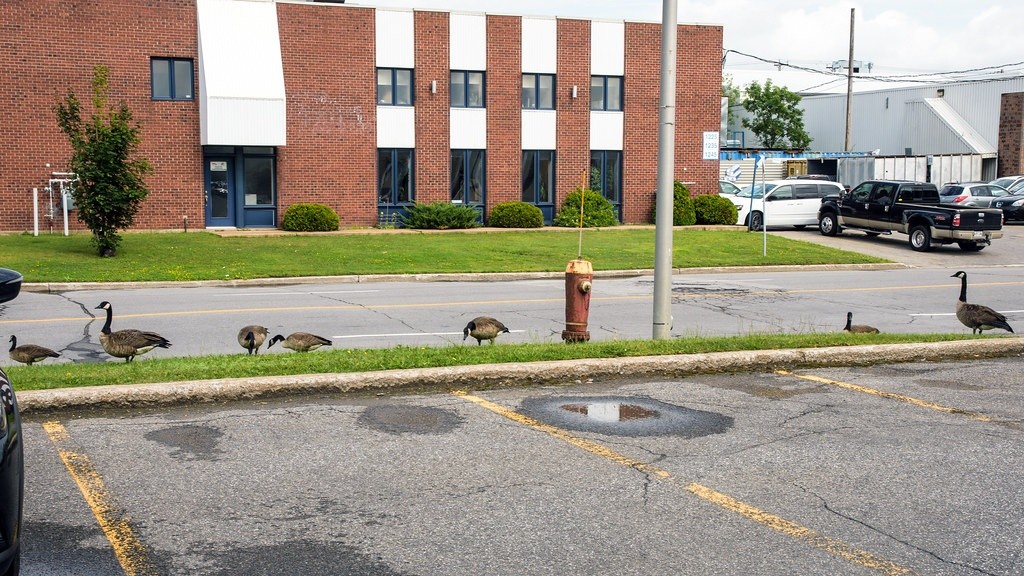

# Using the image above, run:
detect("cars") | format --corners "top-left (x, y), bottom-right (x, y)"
top-left (940, 182), bottom-right (1012, 207)
top-left (0, 266), bottom-right (23, 575)
top-left (990, 176), bottom-right (1024, 189)
top-left (718, 180), bottom-right (743, 199)
top-left (988, 181), bottom-right (1024, 224)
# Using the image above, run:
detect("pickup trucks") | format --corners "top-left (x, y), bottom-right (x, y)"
top-left (796, 175), bottom-right (851, 194)
top-left (817, 180), bottom-right (1004, 252)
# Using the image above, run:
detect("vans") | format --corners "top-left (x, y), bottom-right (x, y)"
top-left (729, 180), bottom-right (848, 232)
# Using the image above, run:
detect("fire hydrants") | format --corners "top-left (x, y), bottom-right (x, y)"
top-left (562, 260), bottom-right (593, 342)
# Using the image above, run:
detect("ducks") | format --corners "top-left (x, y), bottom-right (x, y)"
top-left (267, 331), bottom-right (332, 352)
top-left (844, 312), bottom-right (880, 334)
top-left (950, 270), bottom-right (1016, 334)
top-left (238, 326), bottom-right (270, 355)
top-left (8, 334), bottom-right (61, 366)
top-left (463, 316), bottom-right (510, 346)
top-left (94, 301), bottom-right (172, 363)
